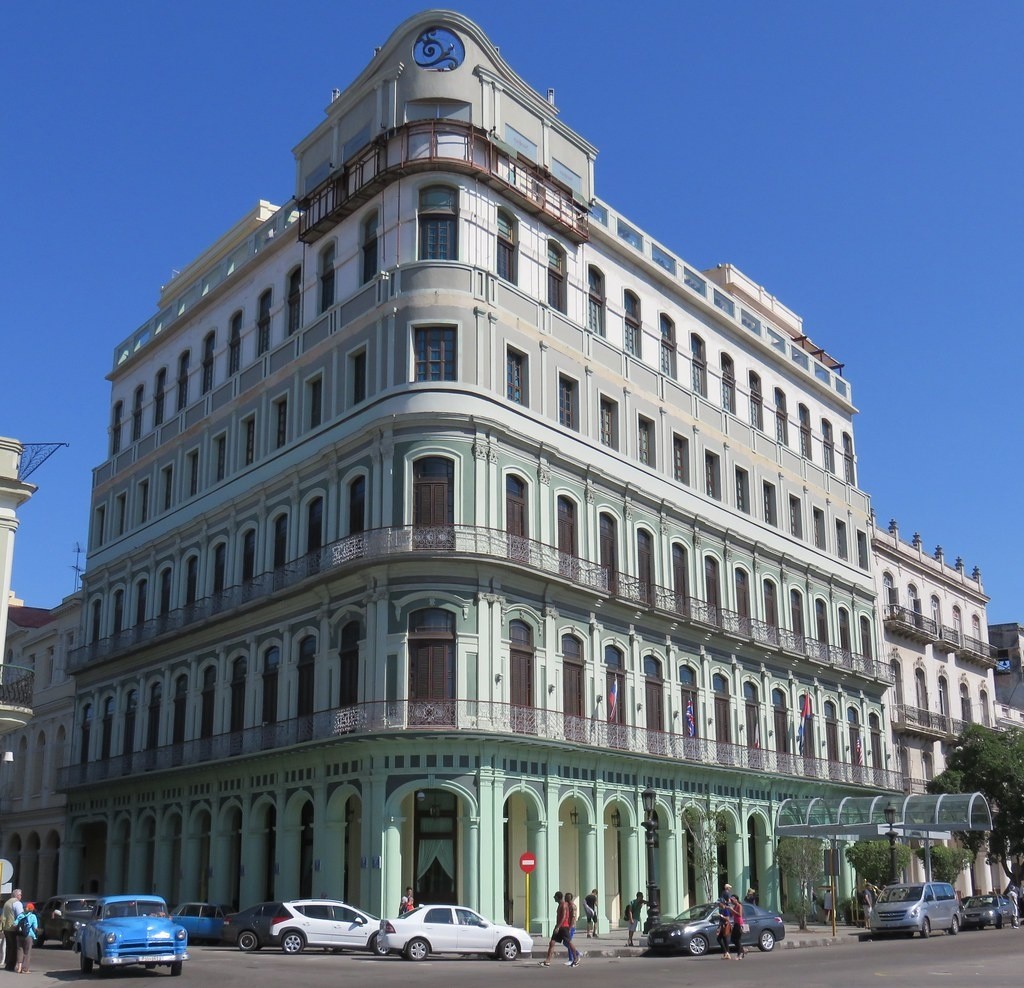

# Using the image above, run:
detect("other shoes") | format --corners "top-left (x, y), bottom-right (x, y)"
top-left (572, 958), bottom-right (581, 967)
top-left (563, 961), bottom-right (574, 966)
top-left (539, 962), bottom-right (551, 968)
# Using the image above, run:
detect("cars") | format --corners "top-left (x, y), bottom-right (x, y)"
top-left (960, 894), bottom-right (1013, 930)
top-left (222, 900), bottom-right (283, 950)
top-left (646, 900), bottom-right (785, 957)
top-left (169, 902), bottom-right (236, 941)
top-left (380, 904), bottom-right (534, 961)
top-left (79, 895), bottom-right (190, 976)
top-left (33, 895), bottom-right (100, 948)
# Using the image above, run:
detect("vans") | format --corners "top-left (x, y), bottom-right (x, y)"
top-left (870, 881), bottom-right (961, 938)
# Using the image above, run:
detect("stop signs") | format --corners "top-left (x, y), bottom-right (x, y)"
top-left (519, 852), bottom-right (537, 872)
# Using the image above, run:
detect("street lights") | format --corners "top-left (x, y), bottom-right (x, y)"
top-left (883, 802), bottom-right (899, 884)
top-left (639, 783), bottom-right (660, 934)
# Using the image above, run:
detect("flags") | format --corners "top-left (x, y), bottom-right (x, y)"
top-left (856, 736), bottom-right (863, 766)
top-left (796, 690), bottom-right (812, 756)
top-left (609, 677), bottom-right (618, 723)
top-left (686, 691), bottom-right (696, 738)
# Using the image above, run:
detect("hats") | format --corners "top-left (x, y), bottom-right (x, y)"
top-left (716, 898), bottom-right (725, 904)
top-left (26, 903), bottom-right (35, 910)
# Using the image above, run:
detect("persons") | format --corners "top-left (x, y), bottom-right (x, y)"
top-left (537, 892), bottom-right (584, 968)
top-left (716, 883), bottom-right (757, 961)
top-left (1007, 880), bottom-right (1024, 929)
top-left (862, 883), bottom-right (874, 930)
top-left (823, 890), bottom-right (834, 925)
top-left (399, 887), bottom-right (414, 915)
top-left (1, 889), bottom-right (24, 972)
top-left (583, 889), bottom-right (600, 939)
top-left (624, 893), bottom-right (651, 947)
top-left (11, 903), bottom-right (40, 974)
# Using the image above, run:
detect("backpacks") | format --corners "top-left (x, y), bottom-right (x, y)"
top-left (624, 905), bottom-right (630, 921)
top-left (16, 917), bottom-right (30, 937)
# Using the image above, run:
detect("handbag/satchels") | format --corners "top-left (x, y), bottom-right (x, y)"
top-left (740, 924), bottom-right (750, 935)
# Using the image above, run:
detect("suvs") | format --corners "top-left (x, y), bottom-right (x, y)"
top-left (269, 897), bottom-right (393, 956)
